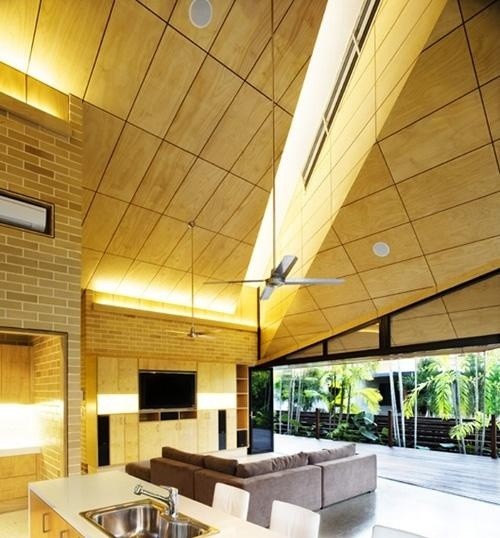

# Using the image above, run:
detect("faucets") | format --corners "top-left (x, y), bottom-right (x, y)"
top-left (133, 481), bottom-right (181, 517)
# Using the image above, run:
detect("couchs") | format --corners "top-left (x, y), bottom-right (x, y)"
top-left (126, 455), bottom-right (377, 528)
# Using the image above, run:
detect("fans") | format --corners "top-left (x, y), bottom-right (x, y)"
top-left (204, 2), bottom-right (347, 301)
top-left (166, 219), bottom-right (222, 340)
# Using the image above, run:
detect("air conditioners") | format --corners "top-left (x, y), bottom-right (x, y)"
top-left (0, 195), bottom-right (47, 231)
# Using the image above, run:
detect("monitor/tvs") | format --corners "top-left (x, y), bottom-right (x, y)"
top-left (139, 370), bottom-right (196, 410)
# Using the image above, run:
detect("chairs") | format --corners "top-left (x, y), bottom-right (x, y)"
top-left (212, 482), bottom-right (250, 521)
top-left (269, 500), bottom-right (320, 538)
top-left (372, 525), bottom-right (425, 538)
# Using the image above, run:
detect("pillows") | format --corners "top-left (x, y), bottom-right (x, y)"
top-left (305, 441), bottom-right (356, 463)
top-left (162, 446), bottom-right (203, 467)
top-left (203, 455), bottom-right (237, 474)
top-left (237, 451), bottom-right (309, 478)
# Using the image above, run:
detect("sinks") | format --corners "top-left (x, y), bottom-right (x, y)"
top-left (84, 497), bottom-right (212, 538)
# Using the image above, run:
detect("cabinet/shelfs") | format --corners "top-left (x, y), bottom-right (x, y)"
top-left (96, 356), bottom-right (249, 465)
top-left (30, 490), bottom-right (83, 537)
